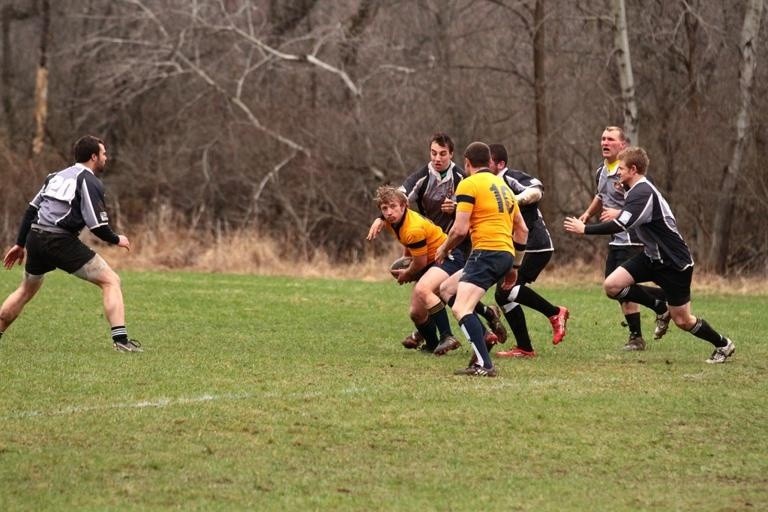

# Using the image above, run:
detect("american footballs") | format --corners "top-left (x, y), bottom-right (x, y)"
top-left (391, 257), bottom-right (411, 279)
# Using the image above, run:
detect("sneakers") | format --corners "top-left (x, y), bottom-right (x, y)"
top-left (113, 339), bottom-right (143, 353)
top-left (455, 363), bottom-right (496, 377)
top-left (402, 332), bottom-right (418, 349)
top-left (549, 306), bottom-right (569, 345)
top-left (654, 302), bottom-right (671, 340)
top-left (468, 330), bottom-right (498, 366)
top-left (421, 344), bottom-right (434, 353)
top-left (705, 337), bottom-right (735, 363)
top-left (496, 345), bottom-right (535, 357)
top-left (621, 337), bottom-right (646, 351)
top-left (433, 336), bottom-right (460, 355)
top-left (486, 305), bottom-right (507, 343)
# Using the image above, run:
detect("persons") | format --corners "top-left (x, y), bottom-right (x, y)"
top-left (434, 142), bottom-right (529, 377)
top-left (576, 125), bottom-right (668, 352)
top-left (373, 185), bottom-right (465, 356)
top-left (563, 144), bottom-right (735, 364)
top-left (487, 144), bottom-right (569, 357)
top-left (0, 135), bottom-right (144, 355)
top-left (365, 134), bottom-right (508, 352)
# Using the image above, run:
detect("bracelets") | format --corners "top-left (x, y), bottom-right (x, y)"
top-left (512, 265), bottom-right (521, 268)
